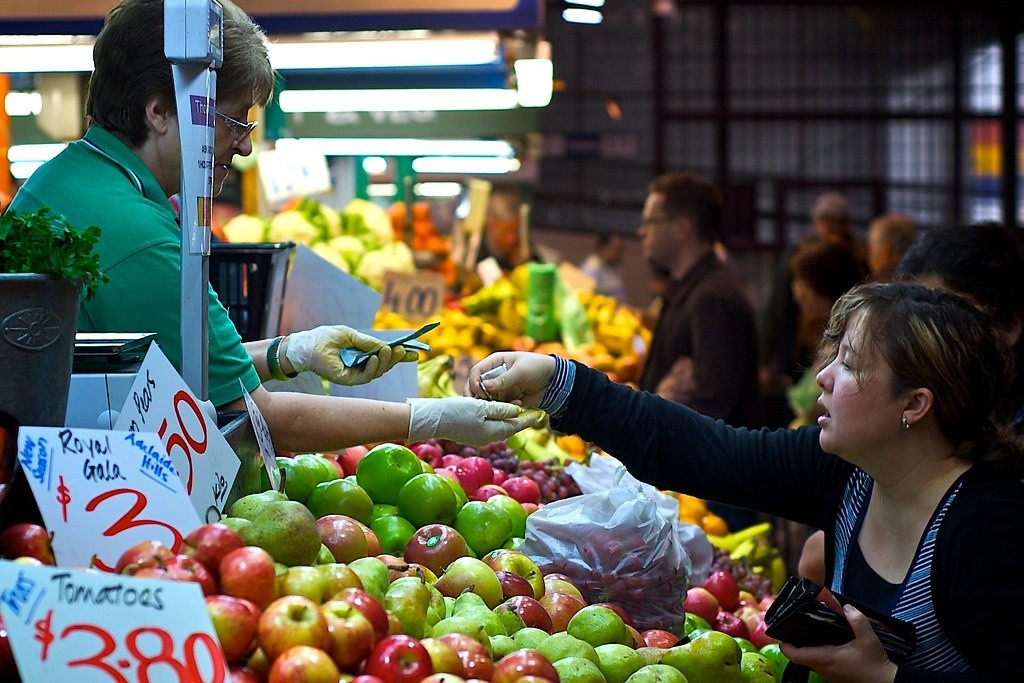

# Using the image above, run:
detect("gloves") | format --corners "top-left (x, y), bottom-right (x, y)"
top-left (284, 324), bottom-right (420, 388)
top-left (403, 395), bottom-right (541, 447)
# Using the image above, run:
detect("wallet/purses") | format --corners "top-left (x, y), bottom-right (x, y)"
top-left (763, 575), bottom-right (917, 666)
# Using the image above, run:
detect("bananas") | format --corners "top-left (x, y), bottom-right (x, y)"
top-left (414, 357), bottom-right (573, 461)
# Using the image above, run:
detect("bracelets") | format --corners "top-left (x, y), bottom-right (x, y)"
top-left (267, 335), bottom-right (297, 382)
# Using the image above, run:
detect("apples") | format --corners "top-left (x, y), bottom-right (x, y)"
top-left (513, 336), bottom-right (644, 388)
top-left (0, 438), bottom-right (559, 683)
top-left (538, 573), bottom-right (827, 683)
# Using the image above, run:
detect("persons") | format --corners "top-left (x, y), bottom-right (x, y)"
top-left (465, 282), bottom-right (1024, 683)
top-left (633, 170), bottom-right (758, 528)
top-left (716, 192), bottom-right (1024, 457)
top-left (0, 0), bottom-right (543, 449)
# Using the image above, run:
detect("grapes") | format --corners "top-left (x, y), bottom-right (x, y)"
top-left (422, 438), bottom-right (773, 639)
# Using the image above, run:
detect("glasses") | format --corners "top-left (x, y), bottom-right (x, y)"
top-left (639, 216), bottom-right (672, 227)
top-left (215, 111), bottom-right (258, 143)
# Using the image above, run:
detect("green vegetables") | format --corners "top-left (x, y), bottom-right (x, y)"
top-left (0, 206), bottom-right (111, 302)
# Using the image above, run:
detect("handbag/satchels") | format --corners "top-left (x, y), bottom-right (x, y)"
top-left (508, 452), bottom-right (693, 639)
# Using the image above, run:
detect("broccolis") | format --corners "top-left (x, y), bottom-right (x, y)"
top-left (224, 195), bottom-right (414, 292)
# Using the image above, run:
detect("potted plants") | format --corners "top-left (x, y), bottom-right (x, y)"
top-left (0, 207), bottom-right (110, 560)
